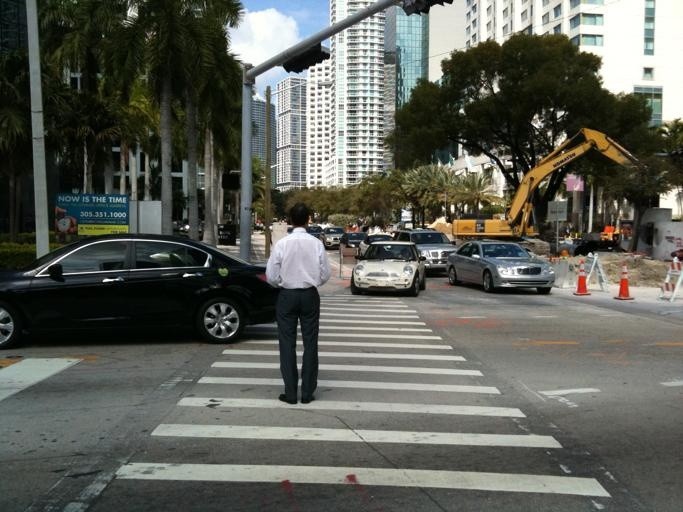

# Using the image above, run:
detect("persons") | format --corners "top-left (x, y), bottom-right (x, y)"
top-left (263, 200), bottom-right (332, 404)
top-left (54, 206), bottom-right (78, 234)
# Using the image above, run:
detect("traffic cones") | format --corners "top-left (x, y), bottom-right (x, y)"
top-left (614, 262), bottom-right (635, 300)
top-left (574, 260), bottom-right (591, 295)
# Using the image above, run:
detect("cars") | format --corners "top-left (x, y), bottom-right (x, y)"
top-left (0, 233), bottom-right (274, 344)
top-left (340, 232), bottom-right (365, 252)
top-left (358, 233), bottom-right (392, 258)
top-left (319, 227), bottom-right (344, 247)
top-left (351, 242), bottom-right (426, 296)
top-left (446, 241), bottom-right (554, 294)
top-left (306, 226), bottom-right (323, 238)
top-left (391, 228), bottom-right (452, 271)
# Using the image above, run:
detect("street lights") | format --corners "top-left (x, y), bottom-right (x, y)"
top-left (403, 0), bottom-right (453, 16)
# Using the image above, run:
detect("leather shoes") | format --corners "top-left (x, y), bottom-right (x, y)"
top-left (301, 397), bottom-right (315, 403)
top-left (279, 394), bottom-right (297, 403)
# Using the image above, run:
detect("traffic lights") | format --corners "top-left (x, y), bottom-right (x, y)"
top-left (281, 43), bottom-right (328, 74)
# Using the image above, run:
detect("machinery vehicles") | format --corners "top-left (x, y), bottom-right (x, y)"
top-left (453, 127), bottom-right (647, 240)
